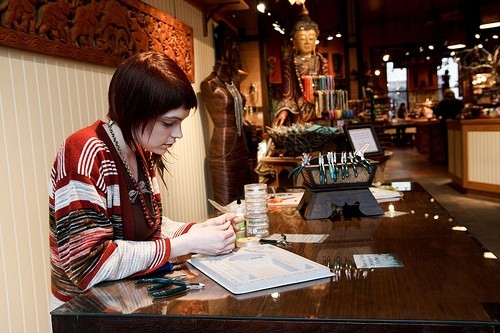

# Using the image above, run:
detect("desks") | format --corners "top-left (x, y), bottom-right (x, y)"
top-left (49, 180), bottom-right (500, 333)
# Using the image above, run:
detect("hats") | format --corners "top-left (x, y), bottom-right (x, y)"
top-left (444, 90), bottom-right (454, 97)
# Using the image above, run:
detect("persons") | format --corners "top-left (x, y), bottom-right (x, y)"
top-left (433, 89), bottom-right (463, 140)
top-left (398, 103), bottom-right (405, 118)
top-left (273, 14), bottom-right (336, 128)
top-left (50, 49), bottom-right (245, 333)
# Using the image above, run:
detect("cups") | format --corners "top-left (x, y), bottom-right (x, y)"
top-left (244, 184), bottom-right (269, 238)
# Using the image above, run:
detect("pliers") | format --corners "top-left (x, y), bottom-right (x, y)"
top-left (135, 273), bottom-right (194, 291)
top-left (135, 278), bottom-right (205, 297)
top-left (260, 232), bottom-right (287, 245)
top-left (288, 151), bottom-right (374, 183)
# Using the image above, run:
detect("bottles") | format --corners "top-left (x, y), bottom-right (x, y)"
top-left (233, 198), bottom-right (246, 237)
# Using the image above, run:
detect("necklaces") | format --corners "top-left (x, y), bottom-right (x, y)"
top-left (109, 124), bottom-right (160, 226)
